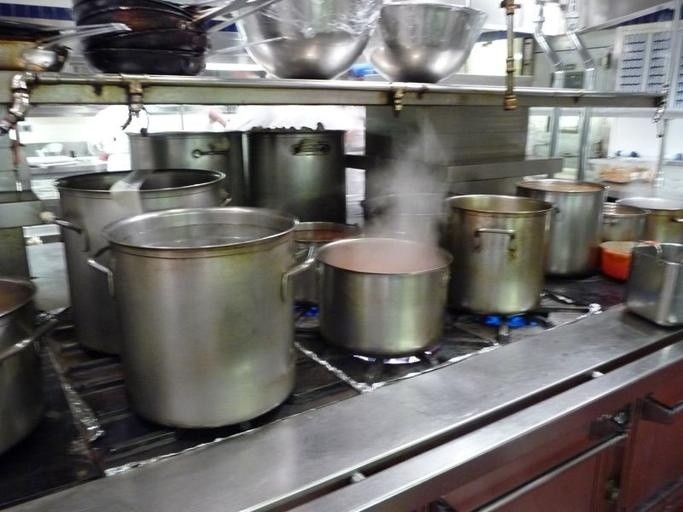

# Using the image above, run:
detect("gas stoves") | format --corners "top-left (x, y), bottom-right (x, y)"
top-left (288, 276), bottom-right (625, 396)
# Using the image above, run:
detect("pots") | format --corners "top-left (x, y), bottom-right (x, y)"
top-left (84, 207), bottom-right (317, 431)
top-left (515, 178), bottom-right (604, 280)
top-left (625, 241), bottom-right (683, 328)
top-left (72, 1), bottom-right (284, 74)
top-left (126, 128), bottom-right (251, 208)
top-left (246, 124), bottom-right (349, 223)
top-left (0, 274), bottom-right (55, 453)
top-left (598, 239), bottom-right (650, 280)
top-left (313, 236), bottom-right (455, 355)
top-left (359, 190), bottom-right (455, 246)
top-left (616, 195), bottom-right (683, 246)
top-left (0, 21), bottom-right (133, 76)
top-left (51, 166), bottom-right (226, 360)
top-left (288, 219), bottom-right (362, 305)
top-left (439, 193), bottom-right (557, 313)
top-left (600, 200), bottom-right (651, 244)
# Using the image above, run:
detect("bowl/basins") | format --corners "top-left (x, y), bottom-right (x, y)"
top-left (225, 0), bottom-right (376, 81)
top-left (369, 0), bottom-right (486, 85)
